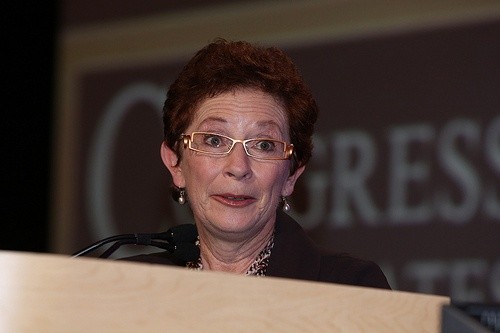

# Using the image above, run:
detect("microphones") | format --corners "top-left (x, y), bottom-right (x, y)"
top-left (100, 239), bottom-right (201, 263)
top-left (69, 224), bottom-right (198, 257)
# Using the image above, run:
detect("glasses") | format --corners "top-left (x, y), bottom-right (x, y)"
top-left (180, 131), bottom-right (297, 160)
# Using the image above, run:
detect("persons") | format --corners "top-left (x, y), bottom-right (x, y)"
top-left (118, 42), bottom-right (392, 292)
